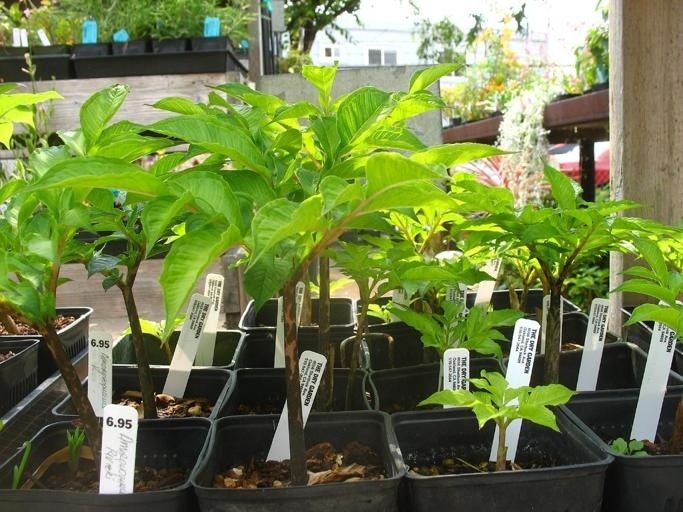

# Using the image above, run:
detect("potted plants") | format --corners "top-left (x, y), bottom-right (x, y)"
top-left (0, 48), bottom-right (682, 511)
